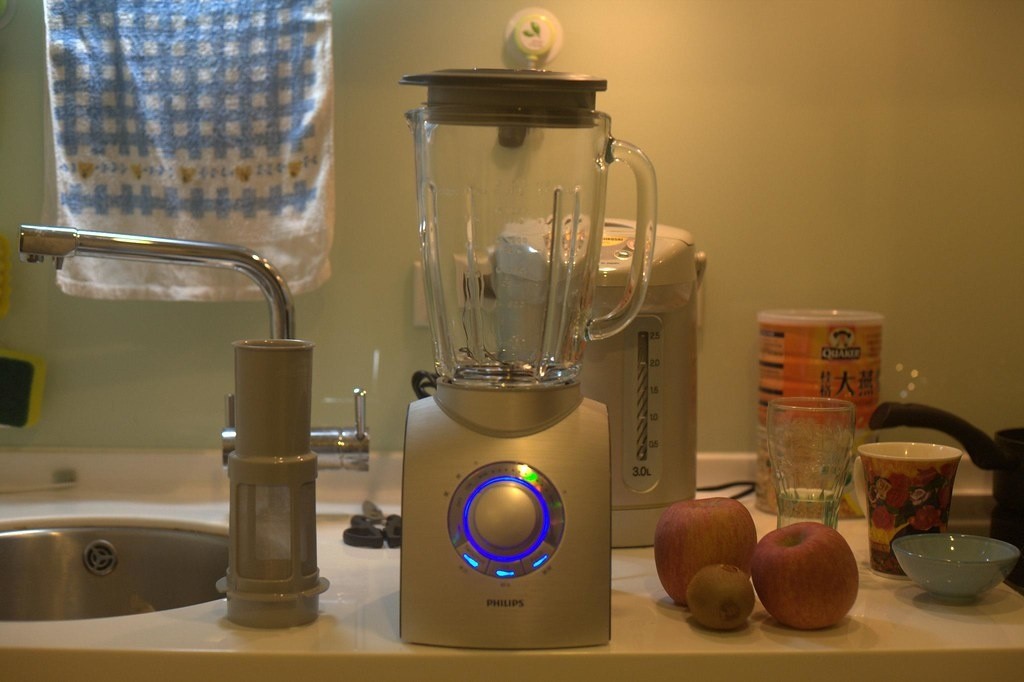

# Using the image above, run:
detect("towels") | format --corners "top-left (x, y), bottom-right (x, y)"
top-left (41, 0), bottom-right (336, 301)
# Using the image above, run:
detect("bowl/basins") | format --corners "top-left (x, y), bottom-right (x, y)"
top-left (891, 534), bottom-right (1019, 605)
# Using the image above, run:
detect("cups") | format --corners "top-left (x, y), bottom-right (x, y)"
top-left (768, 396), bottom-right (856, 530)
top-left (858, 441), bottom-right (963, 580)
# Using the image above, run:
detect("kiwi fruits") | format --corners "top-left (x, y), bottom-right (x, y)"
top-left (685, 564), bottom-right (755, 631)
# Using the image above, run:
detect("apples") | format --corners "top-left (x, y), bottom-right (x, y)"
top-left (755, 521), bottom-right (858, 631)
top-left (655, 497), bottom-right (757, 606)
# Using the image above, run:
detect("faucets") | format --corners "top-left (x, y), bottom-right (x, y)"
top-left (17, 220), bottom-right (330, 628)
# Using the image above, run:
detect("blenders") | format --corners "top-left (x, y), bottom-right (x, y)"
top-left (395, 66), bottom-right (658, 652)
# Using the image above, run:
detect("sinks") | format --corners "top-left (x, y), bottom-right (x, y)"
top-left (0, 513), bottom-right (230, 624)
